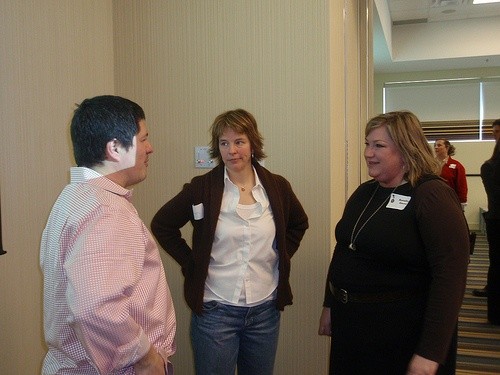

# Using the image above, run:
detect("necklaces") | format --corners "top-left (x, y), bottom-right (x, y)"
top-left (348, 179), bottom-right (403, 252)
top-left (229, 170), bottom-right (253, 192)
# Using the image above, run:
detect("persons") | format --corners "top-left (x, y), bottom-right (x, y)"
top-left (433, 138), bottom-right (468, 213)
top-left (319, 110), bottom-right (470, 375)
top-left (479, 137), bottom-right (500, 325)
top-left (37, 94), bottom-right (178, 374)
top-left (492, 119), bottom-right (500, 143)
top-left (152, 107), bottom-right (311, 375)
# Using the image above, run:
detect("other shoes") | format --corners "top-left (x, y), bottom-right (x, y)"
top-left (473, 287), bottom-right (487, 297)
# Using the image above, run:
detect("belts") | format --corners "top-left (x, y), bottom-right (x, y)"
top-left (327, 279), bottom-right (426, 304)
